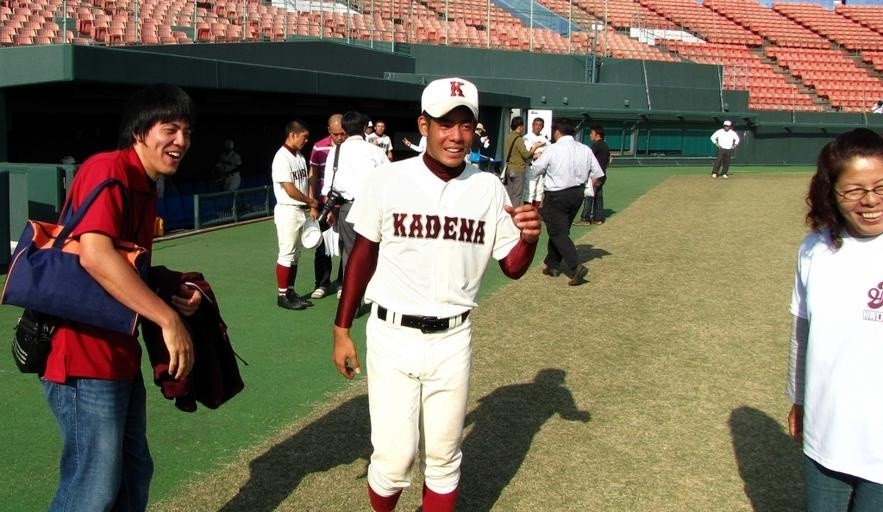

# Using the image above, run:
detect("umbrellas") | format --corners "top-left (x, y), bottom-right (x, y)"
top-left (543, 267), bottom-right (561, 277)
top-left (568, 265), bottom-right (588, 285)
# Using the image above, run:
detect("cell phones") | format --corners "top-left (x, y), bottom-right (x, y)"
top-left (315, 189), bottom-right (343, 227)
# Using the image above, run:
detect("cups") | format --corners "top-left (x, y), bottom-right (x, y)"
top-left (832, 185), bottom-right (883, 200)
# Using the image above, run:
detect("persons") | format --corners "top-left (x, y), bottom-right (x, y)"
top-left (331, 76), bottom-right (541, 510)
top-left (871, 100), bottom-right (883, 114)
top-left (307, 113), bottom-right (347, 300)
top-left (12, 83), bottom-right (203, 512)
top-left (785, 127), bottom-right (882, 511)
top-left (709, 120), bottom-right (740, 178)
top-left (532, 117), bottom-right (606, 286)
top-left (270, 120), bottom-right (324, 311)
top-left (362, 113), bottom-right (552, 210)
top-left (215, 137), bottom-right (243, 191)
top-left (571, 125), bottom-right (612, 225)
top-left (319, 109), bottom-right (391, 318)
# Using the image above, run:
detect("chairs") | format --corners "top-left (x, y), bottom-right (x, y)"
top-left (573, 220), bottom-right (590, 226)
top-left (712, 173), bottom-right (716, 177)
top-left (292, 292), bottom-right (312, 306)
top-left (310, 286), bottom-right (327, 299)
top-left (337, 289), bottom-right (342, 299)
top-left (0, 0), bottom-right (719, 69)
top-left (720, 0), bottom-right (883, 114)
top-left (591, 218), bottom-right (602, 224)
top-left (723, 175), bottom-right (727, 178)
top-left (278, 295), bottom-right (304, 309)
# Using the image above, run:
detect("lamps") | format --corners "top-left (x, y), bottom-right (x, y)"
top-left (720, 0), bottom-right (883, 114)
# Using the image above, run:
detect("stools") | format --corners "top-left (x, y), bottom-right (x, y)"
top-left (377, 306), bottom-right (469, 333)
top-left (298, 205), bottom-right (311, 208)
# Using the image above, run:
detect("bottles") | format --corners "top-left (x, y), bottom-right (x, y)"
top-left (477, 122), bottom-right (486, 130)
top-left (421, 78), bottom-right (479, 122)
top-left (723, 120), bottom-right (731, 126)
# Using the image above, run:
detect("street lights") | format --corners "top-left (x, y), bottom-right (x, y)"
top-left (0, 178), bottom-right (149, 336)
top-left (10, 310), bottom-right (52, 373)
top-left (469, 152), bottom-right (480, 162)
top-left (501, 163), bottom-right (509, 185)
